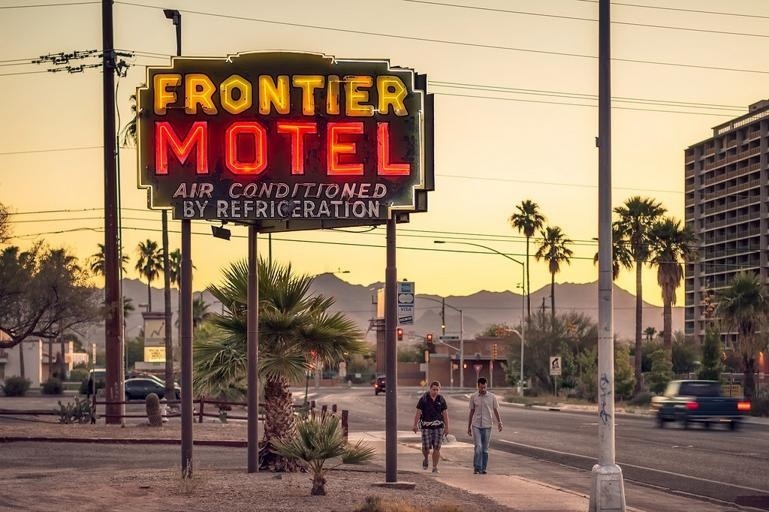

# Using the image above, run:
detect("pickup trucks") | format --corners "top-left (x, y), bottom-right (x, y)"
top-left (648, 377), bottom-right (753, 434)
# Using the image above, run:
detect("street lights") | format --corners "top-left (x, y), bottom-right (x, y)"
top-left (433, 240), bottom-right (525, 398)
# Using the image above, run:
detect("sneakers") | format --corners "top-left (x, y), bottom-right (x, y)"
top-left (423, 460), bottom-right (440, 474)
top-left (475, 470), bottom-right (487, 474)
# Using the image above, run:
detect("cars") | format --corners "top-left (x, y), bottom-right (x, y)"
top-left (374, 374), bottom-right (386, 396)
top-left (87, 368), bottom-right (181, 401)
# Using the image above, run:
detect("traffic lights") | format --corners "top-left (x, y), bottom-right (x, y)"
top-left (426, 333), bottom-right (432, 345)
top-left (397, 327), bottom-right (403, 341)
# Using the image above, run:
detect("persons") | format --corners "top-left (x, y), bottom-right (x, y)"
top-left (466, 377), bottom-right (503, 474)
top-left (412, 380), bottom-right (449, 474)
